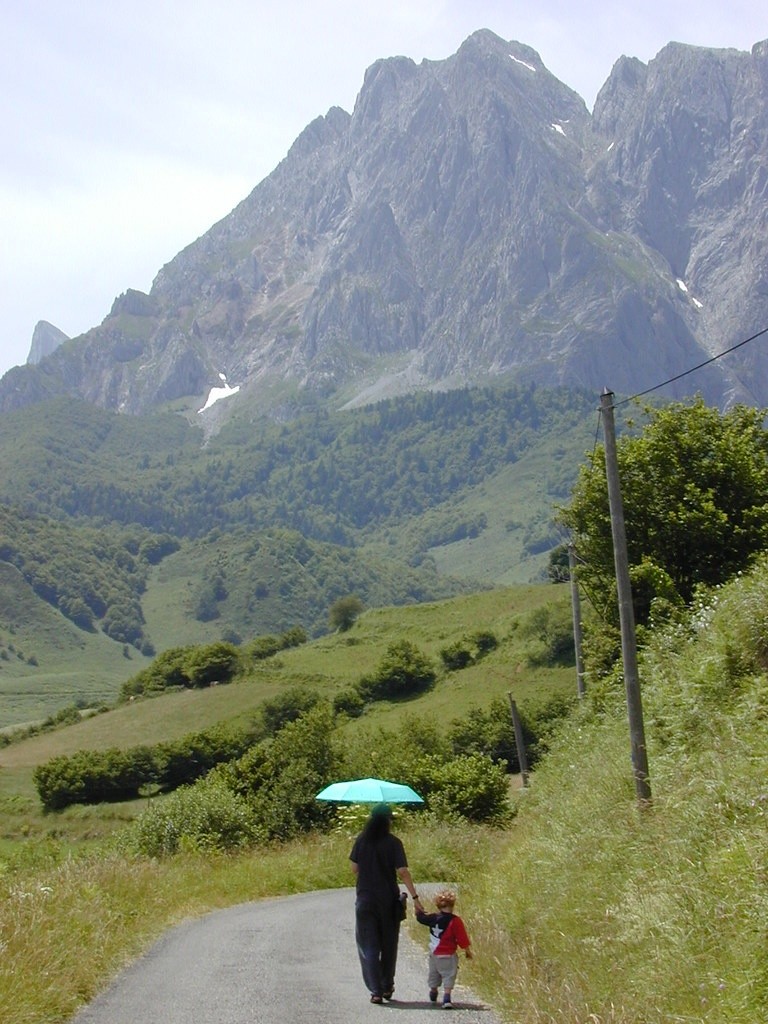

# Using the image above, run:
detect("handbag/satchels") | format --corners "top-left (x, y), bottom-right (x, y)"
top-left (399, 892), bottom-right (407, 920)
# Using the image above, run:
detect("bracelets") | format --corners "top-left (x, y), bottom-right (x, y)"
top-left (413, 895), bottom-right (418, 899)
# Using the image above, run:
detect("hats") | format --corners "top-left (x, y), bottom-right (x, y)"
top-left (372, 804), bottom-right (397, 820)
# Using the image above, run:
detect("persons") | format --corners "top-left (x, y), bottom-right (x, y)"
top-left (349, 805), bottom-right (425, 1003)
top-left (416, 892), bottom-right (473, 1009)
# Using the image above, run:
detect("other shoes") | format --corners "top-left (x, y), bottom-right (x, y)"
top-left (384, 986), bottom-right (395, 999)
top-left (370, 995), bottom-right (382, 1004)
top-left (441, 999), bottom-right (453, 1008)
top-left (430, 990), bottom-right (438, 1003)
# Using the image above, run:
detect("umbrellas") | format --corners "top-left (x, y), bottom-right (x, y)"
top-left (314, 778), bottom-right (425, 803)
